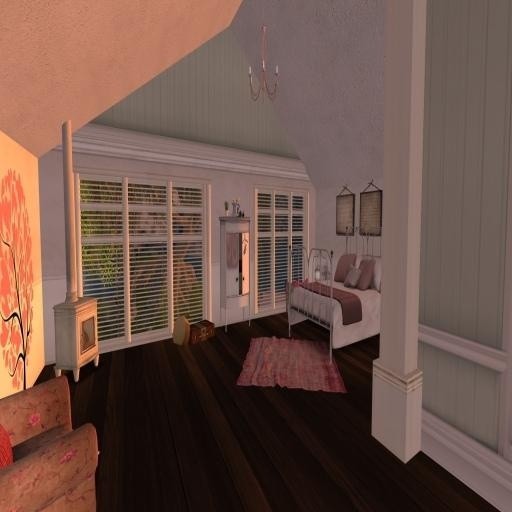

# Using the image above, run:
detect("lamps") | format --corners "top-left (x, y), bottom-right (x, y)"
top-left (248, 24), bottom-right (279, 101)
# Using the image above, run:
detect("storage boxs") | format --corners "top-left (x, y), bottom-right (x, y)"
top-left (190, 320), bottom-right (215, 344)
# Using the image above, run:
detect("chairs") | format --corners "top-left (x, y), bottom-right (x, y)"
top-left (0, 374), bottom-right (99, 512)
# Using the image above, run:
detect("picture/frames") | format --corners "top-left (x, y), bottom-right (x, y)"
top-left (359, 190), bottom-right (383, 236)
top-left (335, 193), bottom-right (355, 236)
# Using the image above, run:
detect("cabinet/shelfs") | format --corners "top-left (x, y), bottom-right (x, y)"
top-left (218, 216), bottom-right (252, 332)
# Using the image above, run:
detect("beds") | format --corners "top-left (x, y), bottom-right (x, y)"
top-left (286, 225), bottom-right (382, 364)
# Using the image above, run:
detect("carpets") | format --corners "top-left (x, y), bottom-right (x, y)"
top-left (237, 336), bottom-right (348, 394)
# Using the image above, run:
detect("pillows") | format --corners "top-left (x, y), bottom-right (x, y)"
top-left (0, 424), bottom-right (14, 468)
top-left (333, 253), bottom-right (381, 293)
